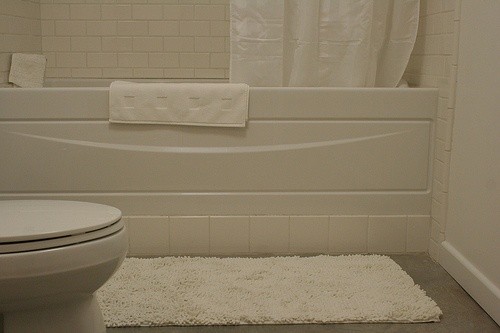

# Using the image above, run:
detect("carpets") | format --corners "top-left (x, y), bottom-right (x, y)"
top-left (94, 253), bottom-right (444, 329)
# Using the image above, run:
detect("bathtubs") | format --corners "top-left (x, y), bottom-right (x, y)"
top-left (0, 78), bottom-right (439, 255)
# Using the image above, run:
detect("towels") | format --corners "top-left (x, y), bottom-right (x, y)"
top-left (9, 53), bottom-right (47, 89)
top-left (109, 80), bottom-right (250, 128)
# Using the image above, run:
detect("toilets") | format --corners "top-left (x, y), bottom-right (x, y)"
top-left (1, 198), bottom-right (129, 333)
